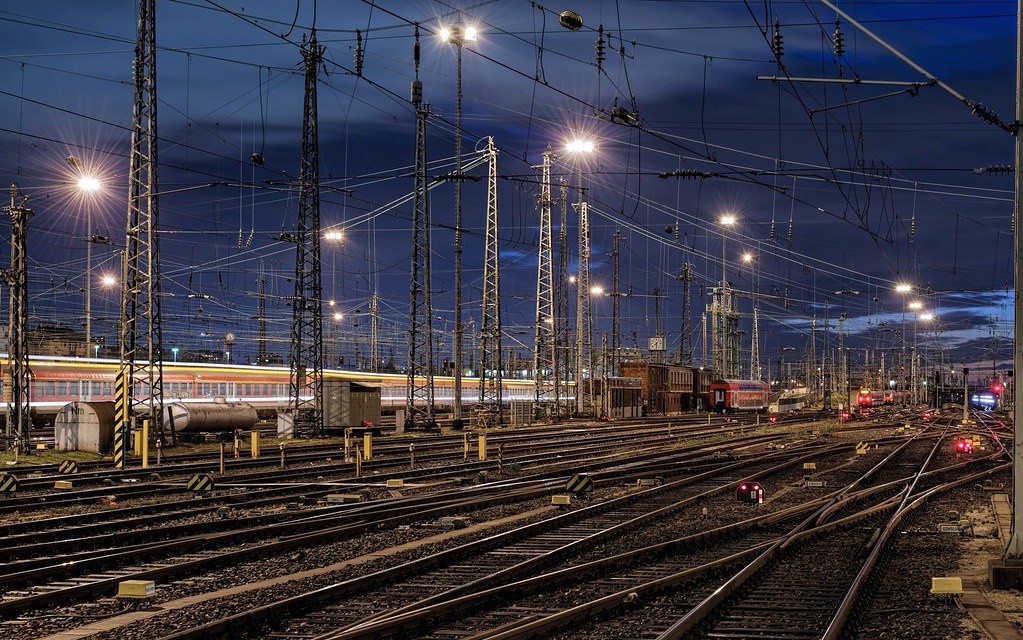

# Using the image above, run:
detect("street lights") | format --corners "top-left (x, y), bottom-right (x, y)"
top-left (717, 214), bottom-right (737, 380)
top-left (896, 282), bottom-right (911, 390)
top-left (920, 313), bottom-right (933, 404)
top-left (438, 11), bottom-right (486, 426)
top-left (562, 138), bottom-right (596, 418)
top-left (324, 229), bottom-right (342, 370)
top-left (908, 300), bottom-right (924, 359)
top-left (78, 175), bottom-right (101, 359)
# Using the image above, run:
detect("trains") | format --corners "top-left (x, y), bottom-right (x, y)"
top-left (0, 352), bottom-right (578, 431)
top-left (708, 378), bottom-right (775, 416)
top-left (857, 388), bottom-right (911, 408)
